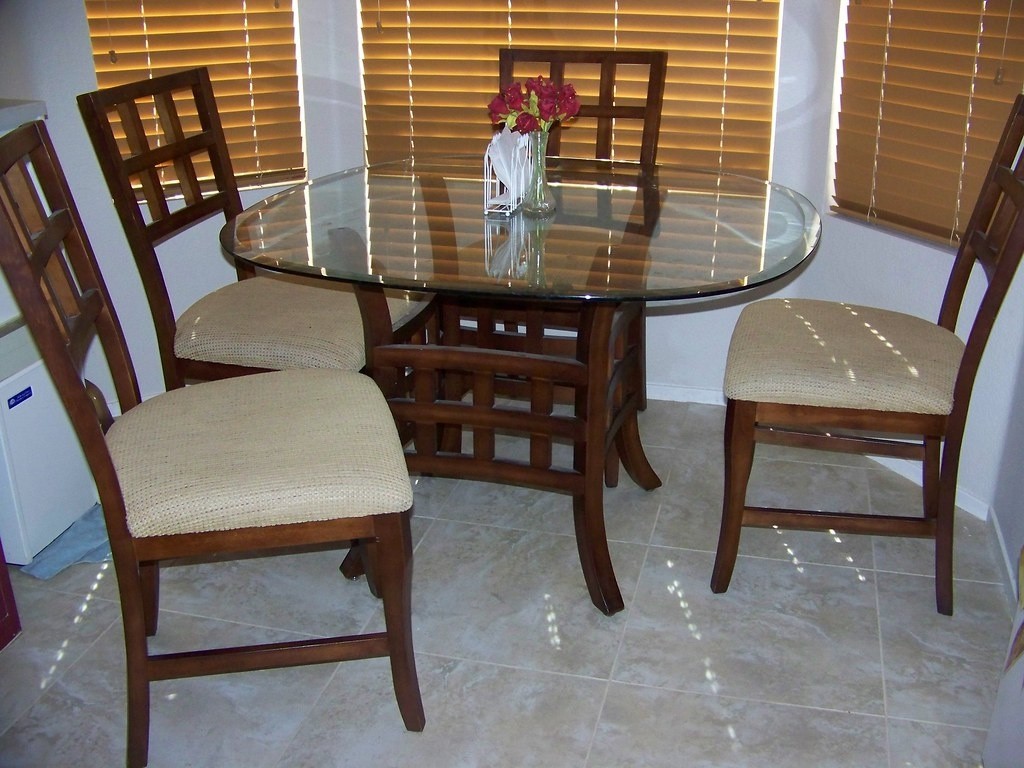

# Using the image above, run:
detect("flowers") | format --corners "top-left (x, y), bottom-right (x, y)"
top-left (488, 73), bottom-right (580, 135)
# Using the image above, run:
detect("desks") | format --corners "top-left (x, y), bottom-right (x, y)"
top-left (216, 152), bottom-right (821, 615)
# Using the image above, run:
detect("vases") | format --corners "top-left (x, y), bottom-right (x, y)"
top-left (519, 131), bottom-right (559, 217)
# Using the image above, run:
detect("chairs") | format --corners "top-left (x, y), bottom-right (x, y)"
top-left (709, 91), bottom-right (1024, 615)
top-left (75, 66), bottom-right (439, 394)
top-left (0, 117), bottom-right (425, 767)
top-left (459, 47), bottom-right (669, 487)
